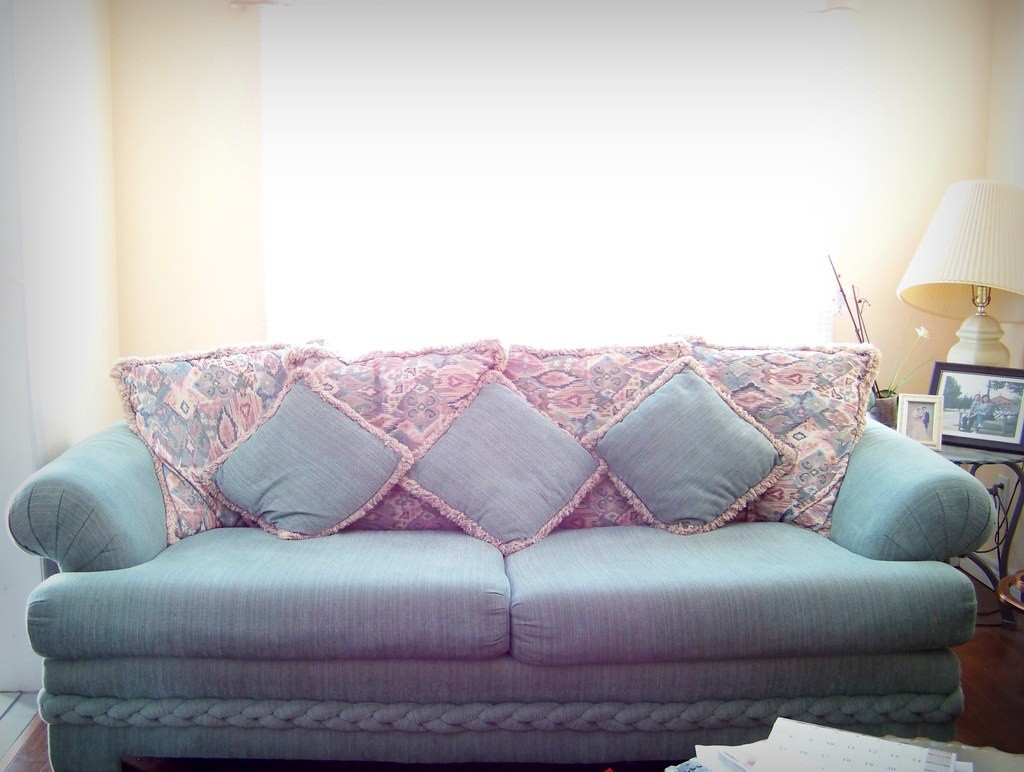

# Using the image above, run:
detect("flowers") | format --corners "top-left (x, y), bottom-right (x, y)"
top-left (827, 254), bottom-right (935, 398)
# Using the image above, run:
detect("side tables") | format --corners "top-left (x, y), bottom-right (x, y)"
top-left (919, 438), bottom-right (1024, 634)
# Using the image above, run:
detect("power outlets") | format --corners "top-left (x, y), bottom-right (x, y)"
top-left (995, 473), bottom-right (1010, 500)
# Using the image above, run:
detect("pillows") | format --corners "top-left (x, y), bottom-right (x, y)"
top-left (112, 340), bottom-right (332, 540)
top-left (285, 338), bottom-right (511, 533)
top-left (502, 339), bottom-right (701, 535)
top-left (675, 341), bottom-right (883, 539)
top-left (587, 355), bottom-right (800, 529)
top-left (407, 370), bottom-right (602, 554)
top-left (199, 362), bottom-right (407, 542)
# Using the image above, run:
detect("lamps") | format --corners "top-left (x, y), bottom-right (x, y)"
top-left (894, 177), bottom-right (1024, 364)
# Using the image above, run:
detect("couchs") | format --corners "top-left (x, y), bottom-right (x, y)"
top-left (7, 338), bottom-right (993, 765)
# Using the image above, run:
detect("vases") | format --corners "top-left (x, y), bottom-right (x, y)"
top-left (869, 397), bottom-right (898, 430)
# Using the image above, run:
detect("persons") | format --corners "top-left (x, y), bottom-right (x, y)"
top-left (961, 394), bottom-right (995, 432)
top-left (912, 407), bottom-right (929, 441)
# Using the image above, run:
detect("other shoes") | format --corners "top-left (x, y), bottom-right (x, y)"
top-left (978, 424), bottom-right (983, 427)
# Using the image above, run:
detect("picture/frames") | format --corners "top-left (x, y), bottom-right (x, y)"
top-left (897, 393), bottom-right (945, 452)
top-left (929, 361), bottom-right (1024, 455)
